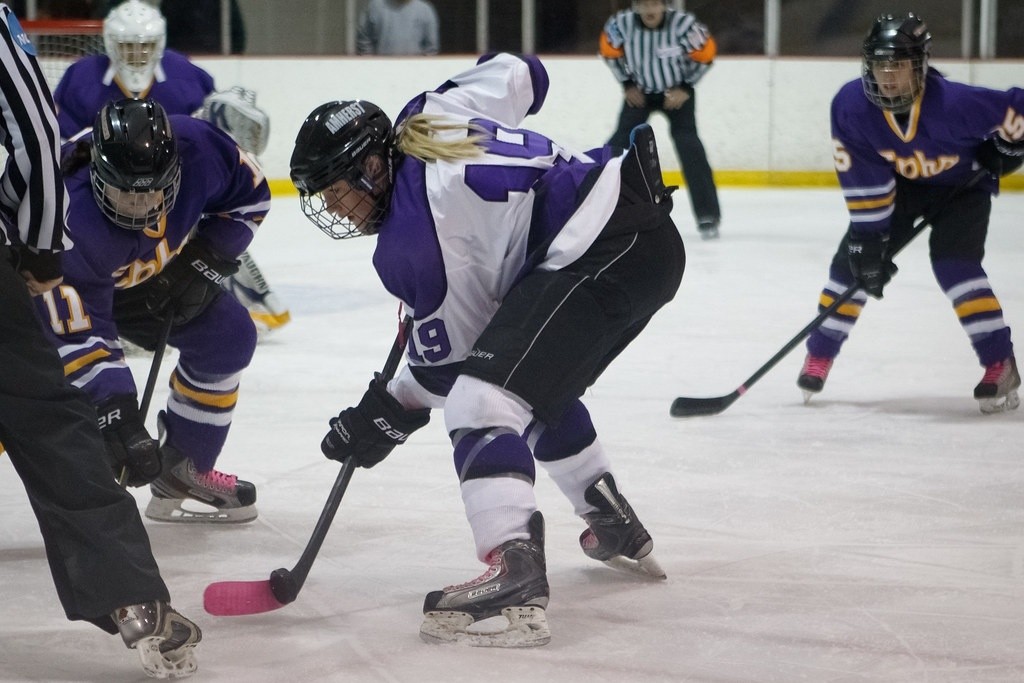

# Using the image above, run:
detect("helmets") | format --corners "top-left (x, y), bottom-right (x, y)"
top-left (290, 99), bottom-right (394, 240)
top-left (89, 98), bottom-right (181, 231)
top-left (102, 0), bottom-right (167, 94)
top-left (861, 11), bottom-right (934, 114)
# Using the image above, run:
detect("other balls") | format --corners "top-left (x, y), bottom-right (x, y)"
top-left (270, 568), bottom-right (299, 604)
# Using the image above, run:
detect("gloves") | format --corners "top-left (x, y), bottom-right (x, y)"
top-left (94, 397), bottom-right (163, 488)
top-left (972, 129), bottom-right (1024, 178)
top-left (662, 89), bottom-right (688, 110)
top-left (145, 237), bottom-right (243, 325)
top-left (625, 85), bottom-right (645, 109)
top-left (847, 232), bottom-right (898, 300)
top-left (320, 369), bottom-right (432, 467)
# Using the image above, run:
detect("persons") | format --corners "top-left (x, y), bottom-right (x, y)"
top-left (0, 2), bottom-right (201, 680)
top-left (356, 0), bottom-right (439, 57)
top-left (33, 98), bottom-right (271, 523)
top-left (797, 11), bottom-right (1024, 416)
top-left (53, 0), bottom-right (292, 358)
top-left (599, 0), bottom-right (720, 241)
top-left (289, 52), bottom-right (686, 649)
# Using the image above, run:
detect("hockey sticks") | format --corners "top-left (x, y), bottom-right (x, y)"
top-left (112, 318), bottom-right (173, 491)
top-left (204, 316), bottom-right (414, 615)
top-left (669, 166), bottom-right (994, 418)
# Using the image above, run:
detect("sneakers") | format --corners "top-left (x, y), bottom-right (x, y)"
top-left (698, 216), bottom-right (718, 240)
top-left (973, 351), bottom-right (1021, 415)
top-left (797, 352), bottom-right (834, 406)
top-left (418, 510), bottom-right (550, 647)
top-left (144, 408), bottom-right (257, 523)
top-left (580, 471), bottom-right (667, 580)
top-left (110, 600), bottom-right (202, 679)
